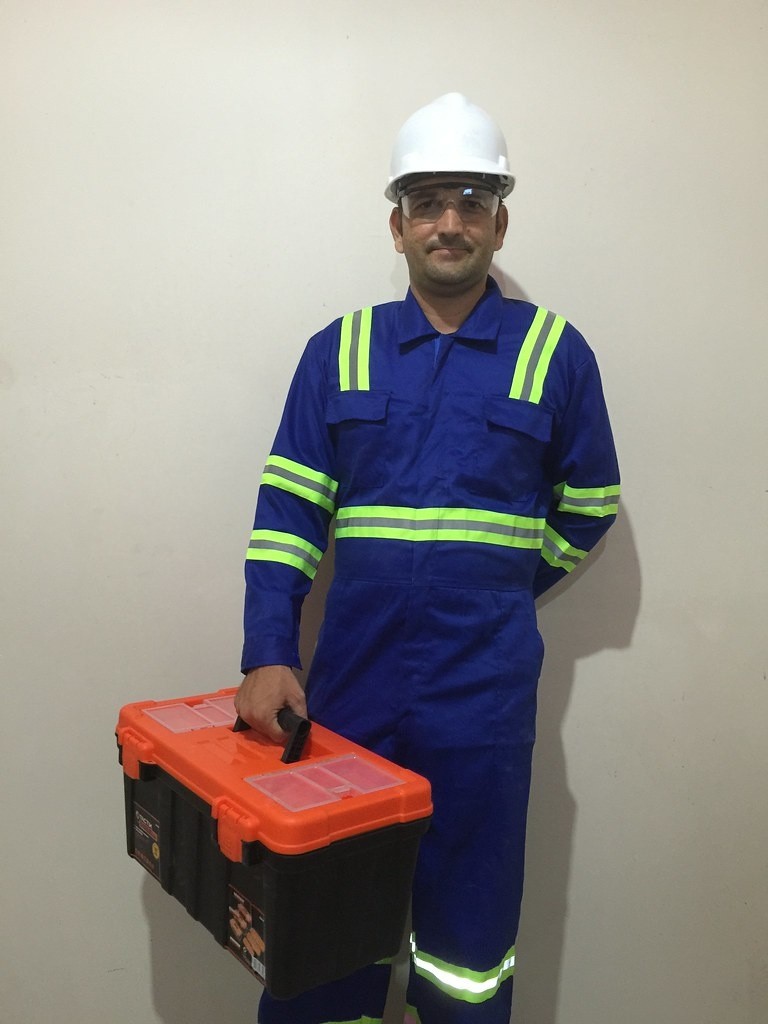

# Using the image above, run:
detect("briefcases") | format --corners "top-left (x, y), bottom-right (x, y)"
top-left (114, 681), bottom-right (436, 1001)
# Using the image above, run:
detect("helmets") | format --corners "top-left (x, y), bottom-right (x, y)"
top-left (384, 92), bottom-right (515, 205)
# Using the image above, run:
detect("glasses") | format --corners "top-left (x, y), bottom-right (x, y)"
top-left (397, 184), bottom-right (501, 222)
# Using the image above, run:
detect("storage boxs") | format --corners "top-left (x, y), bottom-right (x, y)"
top-left (114, 688), bottom-right (434, 1002)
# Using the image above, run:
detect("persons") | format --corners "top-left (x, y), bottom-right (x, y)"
top-left (234, 92), bottom-right (621, 1024)
